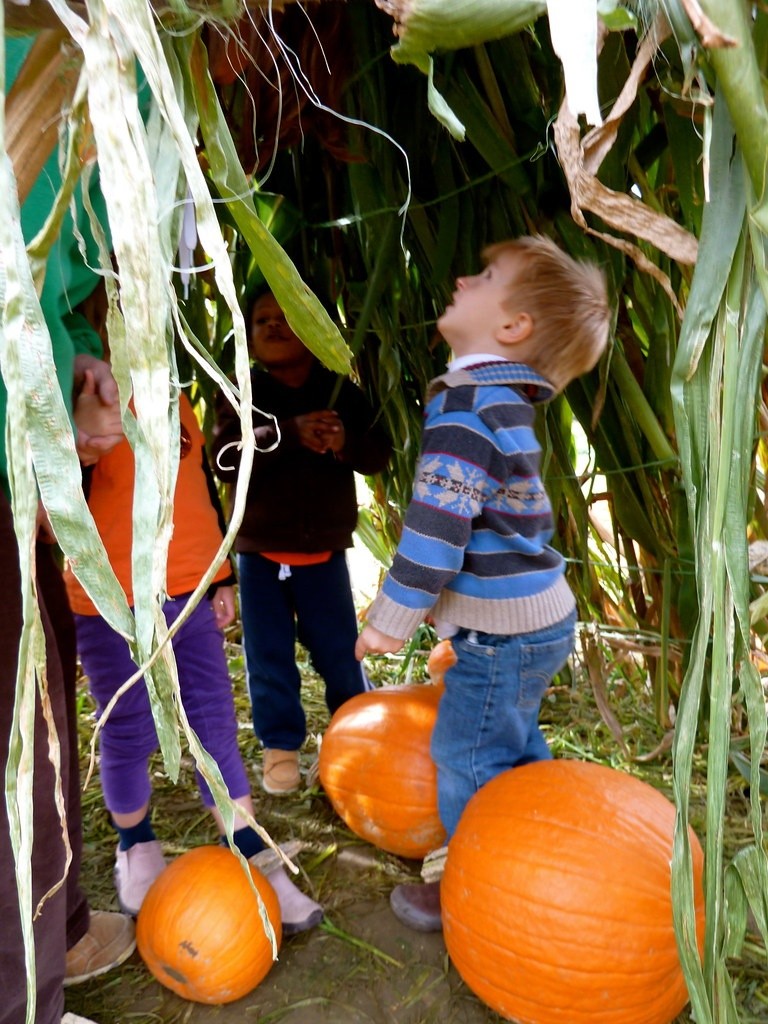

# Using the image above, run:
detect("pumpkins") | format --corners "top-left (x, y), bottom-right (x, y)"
top-left (429, 640), bottom-right (471, 685)
top-left (136, 837), bottom-right (304, 1005)
top-left (320, 685), bottom-right (475, 858)
top-left (447, 760), bottom-right (707, 1024)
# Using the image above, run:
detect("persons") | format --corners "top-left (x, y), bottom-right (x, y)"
top-left (353, 233), bottom-right (613, 935)
top-left (213, 273), bottom-right (398, 799)
top-left (0, 0), bottom-right (160, 1024)
top-left (35, 232), bottom-right (325, 936)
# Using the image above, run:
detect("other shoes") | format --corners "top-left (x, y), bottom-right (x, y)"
top-left (62, 910), bottom-right (135, 989)
top-left (389, 882), bottom-right (442, 931)
top-left (114, 839), bottom-right (167, 917)
top-left (264, 866), bottom-right (325, 932)
top-left (261, 748), bottom-right (301, 799)
